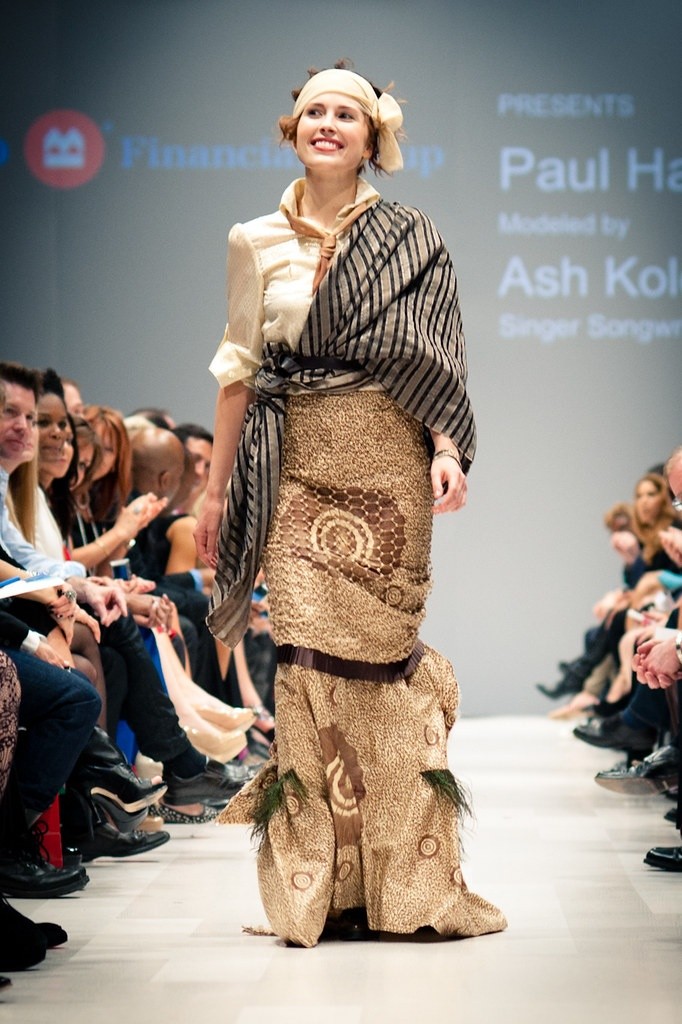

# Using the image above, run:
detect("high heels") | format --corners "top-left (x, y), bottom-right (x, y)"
top-left (86, 760), bottom-right (168, 833)
top-left (538, 677), bottom-right (583, 702)
top-left (177, 697), bottom-right (258, 764)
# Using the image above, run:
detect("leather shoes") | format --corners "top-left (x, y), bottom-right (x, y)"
top-left (81, 822), bottom-right (171, 862)
top-left (0, 818), bottom-right (88, 898)
top-left (574, 717), bottom-right (657, 752)
top-left (163, 755), bottom-right (262, 805)
top-left (643, 847), bottom-right (682, 871)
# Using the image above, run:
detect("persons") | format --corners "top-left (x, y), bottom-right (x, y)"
top-left (193, 55), bottom-right (508, 950)
top-left (536, 443), bottom-right (682, 873)
top-left (1, 360), bottom-right (278, 990)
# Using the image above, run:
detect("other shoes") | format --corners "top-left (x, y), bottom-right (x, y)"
top-left (553, 692), bottom-right (600, 719)
top-left (148, 794), bottom-right (220, 822)
top-left (594, 746), bottom-right (681, 794)
top-left (0, 899), bottom-right (68, 974)
top-left (226, 705), bottom-right (278, 781)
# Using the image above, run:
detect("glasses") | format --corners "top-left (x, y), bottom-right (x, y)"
top-left (672, 498), bottom-right (682, 510)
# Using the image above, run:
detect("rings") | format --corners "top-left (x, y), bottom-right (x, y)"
top-left (62, 588), bottom-right (77, 602)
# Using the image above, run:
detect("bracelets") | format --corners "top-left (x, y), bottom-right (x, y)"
top-left (676, 632), bottom-right (682, 664)
top-left (433, 448), bottom-right (461, 469)
